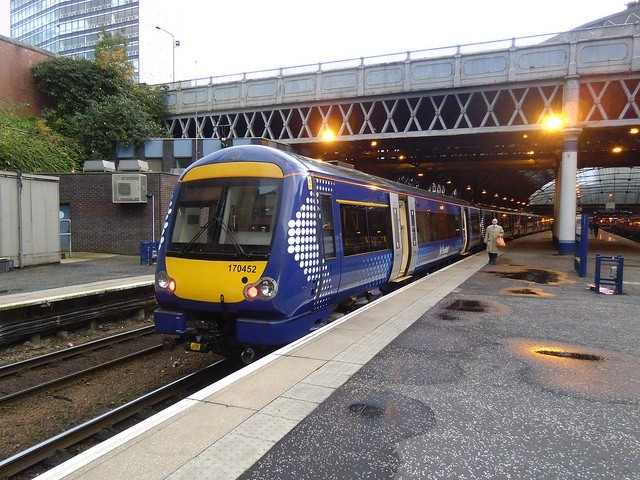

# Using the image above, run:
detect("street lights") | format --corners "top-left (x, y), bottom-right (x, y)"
top-left (155, 26), bottom-right (175, 82)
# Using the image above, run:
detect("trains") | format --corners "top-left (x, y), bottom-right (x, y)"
top-left (153, 143), bottom-right (553, 370)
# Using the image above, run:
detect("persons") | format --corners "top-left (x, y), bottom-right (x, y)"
top-left (483, 218), bottom-right (504, 265)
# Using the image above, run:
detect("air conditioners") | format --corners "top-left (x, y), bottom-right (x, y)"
top-left (111, 173), bottom-right (149, 204)
